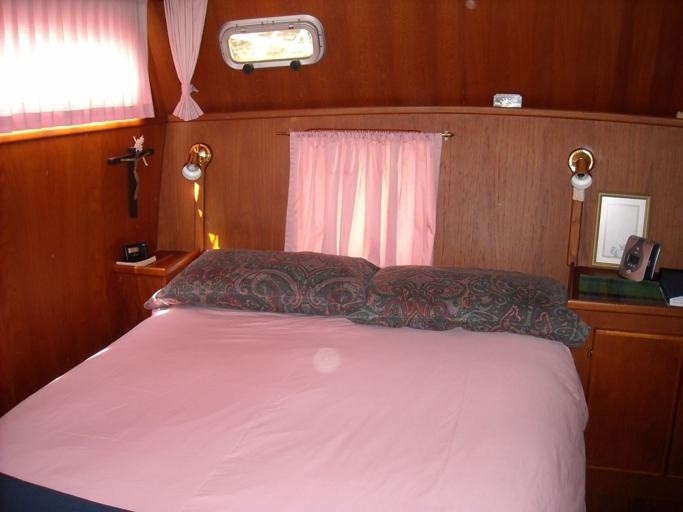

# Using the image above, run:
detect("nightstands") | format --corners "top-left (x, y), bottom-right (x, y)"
top-left (113, 248), bottom-right (199, 330)
top-left (566, 261), bottom-right (682, 504)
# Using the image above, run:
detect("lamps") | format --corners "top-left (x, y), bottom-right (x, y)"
top-left (568, 147), bottom-right (596, 192)
top-left (182, 144), bottom-right (212, 183)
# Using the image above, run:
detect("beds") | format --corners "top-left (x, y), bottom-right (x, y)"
top-left (0, 242), bottom-right (591, 512)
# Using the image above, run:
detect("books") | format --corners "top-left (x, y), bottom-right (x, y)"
top-left (659, 265), bottom-right (683, 306)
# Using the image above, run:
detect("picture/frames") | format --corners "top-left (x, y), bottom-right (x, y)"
top-left (590, 191), bottom-right (654, 270)
